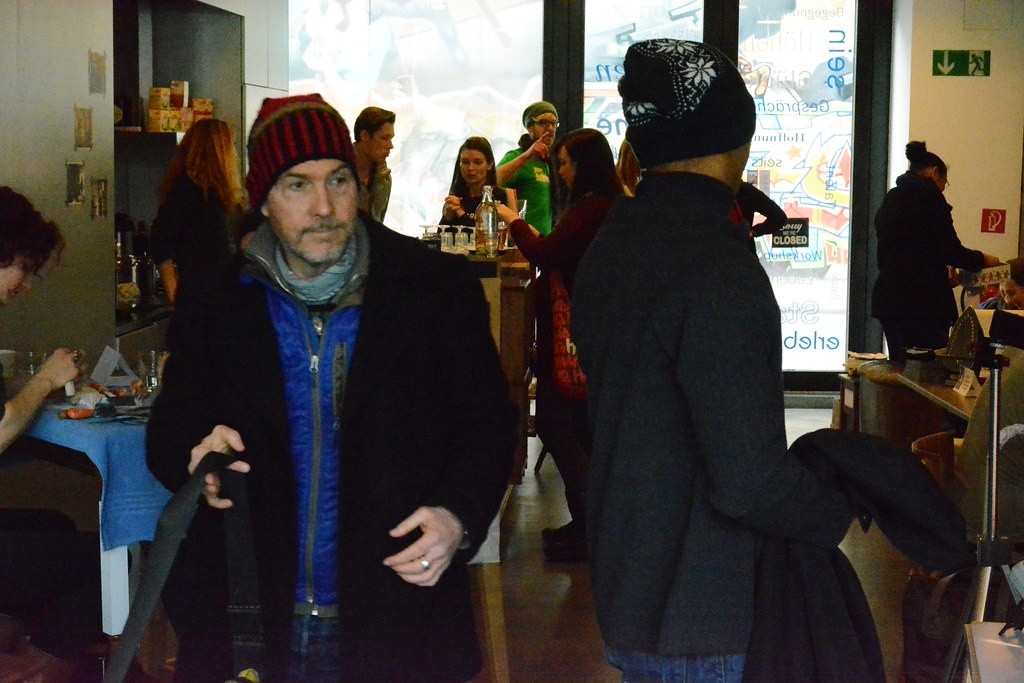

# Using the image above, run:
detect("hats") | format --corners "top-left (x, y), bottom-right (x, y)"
top-left (523, 101), bottom-right (558, 129)
top-left (246, 93), bottom-right (362, 215)
top-left (617, 39), bottom-right (756, 170)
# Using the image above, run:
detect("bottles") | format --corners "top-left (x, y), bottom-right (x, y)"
top-left (474, 183), bottom-right (499, 256)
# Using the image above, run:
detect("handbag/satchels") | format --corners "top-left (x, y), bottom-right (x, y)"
top-left (97, 450), bottom-right (270, 683)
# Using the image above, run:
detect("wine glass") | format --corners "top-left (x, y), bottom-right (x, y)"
top-left (420, 222), bottom-right (475, 251)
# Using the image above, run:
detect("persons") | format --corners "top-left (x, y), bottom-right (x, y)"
top-left (582, 36), bottom-right (872, 683)
top-left (1, 186), bottom-right (84, 457)
top-left (1001, 259), bottom-right (1024, 309)
top-left (493, 101), bottom-right (569, 237)
top-left (436, 136), bottom-right (512, 247)
top-left (874, 140), bottom-right (1001, 365)
top-left (347, 105), bottom-right (397, 224)
top-left (955, 338), bottom-right (1024, 598)
top-left (150, 116), bottom-right (261, 310)
top-left (494, 128), bottom-right (629, 558)
top-left (149, 95), bottom-right (515, 682)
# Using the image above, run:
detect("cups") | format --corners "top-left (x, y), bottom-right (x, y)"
top-left (143, 350), bottom-right (166, 388)
top-left (508, 198), bottom-right (527, 221)
top-left (497, 221), bottom-right (506, 249)
top-left (11, 350), bottom-right (47, 382)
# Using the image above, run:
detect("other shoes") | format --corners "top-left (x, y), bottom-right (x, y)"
top-left (71, 635), bottom-right (162, 683)
top-left (542, 519), bottom-right (587, 559)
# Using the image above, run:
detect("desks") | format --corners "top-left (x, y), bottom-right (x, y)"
top-left (21, 395), bottom-right (179, 683)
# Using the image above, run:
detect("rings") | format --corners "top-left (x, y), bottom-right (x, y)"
top-left (417, 556), bottom-right (431, 573)
top-left (74, 349), bottom-right (82, 358)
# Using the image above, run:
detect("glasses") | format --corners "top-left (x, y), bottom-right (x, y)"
top-left (939, 174), bottom-right (950, 188)
top-left (533, 119), bottom-right (560, 128)
